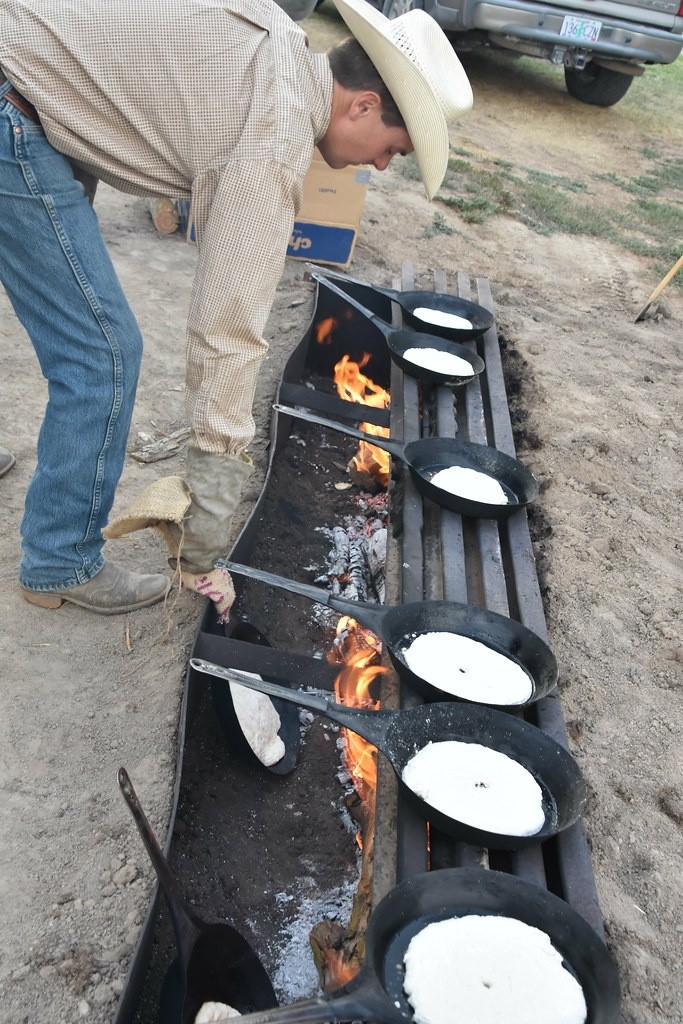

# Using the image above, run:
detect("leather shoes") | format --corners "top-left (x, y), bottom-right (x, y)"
top-left (18, 560), bottom-right (171, 615)
top-left (0, 447), bottom-right (16, 477)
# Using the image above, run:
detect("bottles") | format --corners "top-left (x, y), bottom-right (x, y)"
top-left (146, 197), bottom-right (179, 233)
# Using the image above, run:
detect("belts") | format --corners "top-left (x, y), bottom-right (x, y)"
top-left (0, 69), bottom-right (42, 125)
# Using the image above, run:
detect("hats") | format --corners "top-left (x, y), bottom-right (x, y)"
top-left (333, 0), bottom-right (474, 203)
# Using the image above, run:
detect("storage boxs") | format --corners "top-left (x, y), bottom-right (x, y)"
top-left (175, 144), bottom-right (372, 271)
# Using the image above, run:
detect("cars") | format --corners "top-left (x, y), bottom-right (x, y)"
top-left (370, 1), bottom-right (683, 108)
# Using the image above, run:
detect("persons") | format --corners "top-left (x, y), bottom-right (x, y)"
top-left (1, 0), bottom-right (474, 615)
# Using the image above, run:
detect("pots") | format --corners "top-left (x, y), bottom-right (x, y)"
top-left (116, 765), bottom-right (278, 1024)
top-left (221, 613), bottom-right (301, 775)
top-left (309, 269), bottom-right (485, 387)
top-left (271, 404), bottom-right (539, 520)
top-left (190, 658), bottom-right (589, 851)
top-left (305, 262), bottom-right (495, 343)
top-left (213, 559), bottom-right (559, 717)
top-left (203, 868), bottom-right (622, 1024)
